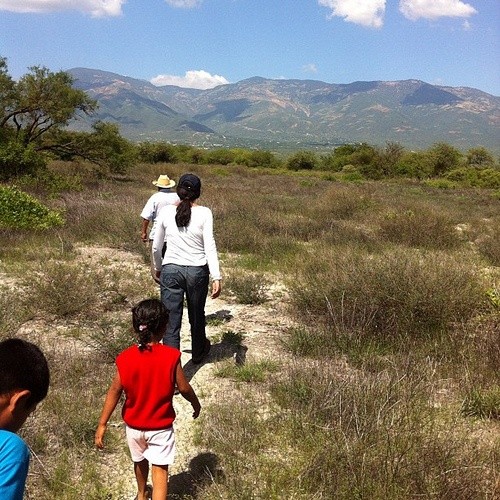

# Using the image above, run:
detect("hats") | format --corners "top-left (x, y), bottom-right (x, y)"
top-left (178, 174), bottom-right (201, 190)
top-left (152, 174), bottom-right (175, 188)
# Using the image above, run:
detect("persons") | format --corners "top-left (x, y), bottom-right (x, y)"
top-left (139, 175), bottom-right (181, 283)
top-left (0, 337), bottom-right (54, 500)
top-left (152, 174), bottom-right (223, 393)
top-left (94, 299), bottom-right (202, 500)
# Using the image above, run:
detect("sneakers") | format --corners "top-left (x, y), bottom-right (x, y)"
top-left (192, 339), bottom-right (210, 364)
